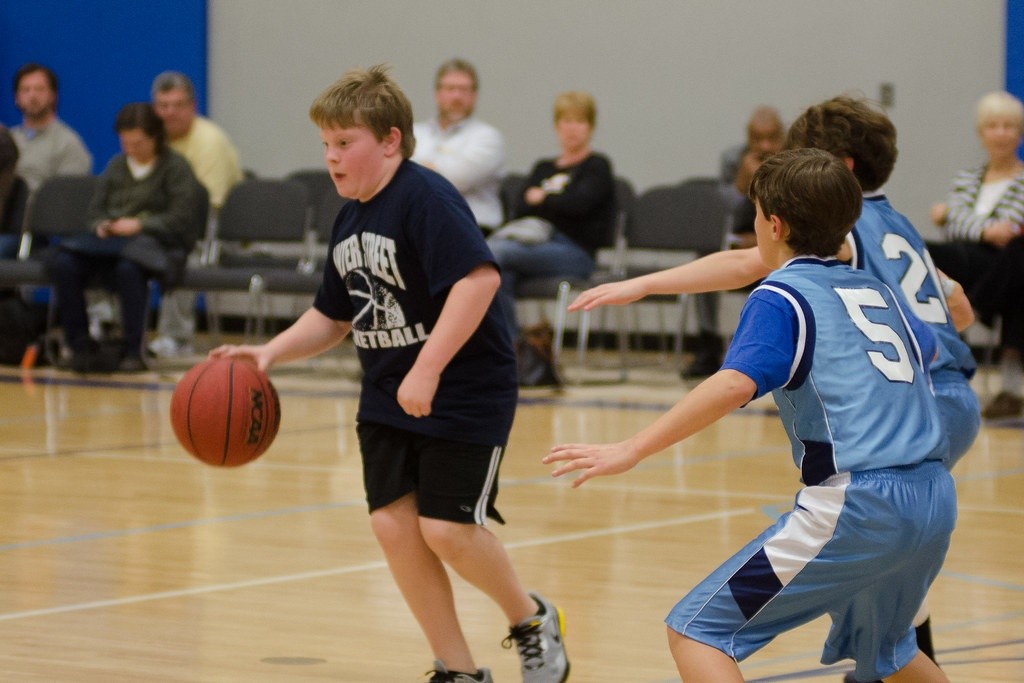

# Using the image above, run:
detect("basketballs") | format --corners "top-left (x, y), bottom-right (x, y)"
top-left (170, 357), bottom-right (280, 467)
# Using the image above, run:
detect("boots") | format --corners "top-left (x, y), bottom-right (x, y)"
top-left (681, 331), bottom-right (722, 380)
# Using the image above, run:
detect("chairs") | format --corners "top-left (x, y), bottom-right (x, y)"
top-left (0, 166), bottom-right (727, 387)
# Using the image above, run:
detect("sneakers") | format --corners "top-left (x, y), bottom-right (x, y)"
top-left (424, 658), bottom-right (493, 683)
top-left (501, 591), bottom-right (571, 683)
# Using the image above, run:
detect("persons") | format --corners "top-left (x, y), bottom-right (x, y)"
top-left (0, 63), bottom-right (249, 373)
top-left (681, 107), bottom-right (790, 379)
top-left (483, 93), bottom-right (617, 390)
top-left (540, 147), bottom-right (959, 683)
top-left (407, 59), bottom-right (508, 235)
top-left (208, 64), bottom-right (571, 683)
top-left (921, 94), bottom-right (1024, 424)
top-left (566, 97), bottom-right (981, 683)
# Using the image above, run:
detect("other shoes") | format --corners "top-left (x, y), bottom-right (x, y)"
top-left (114, 352), bottom-right (145, 372)
top-left (56, 350), bottom-right (105, 373)
